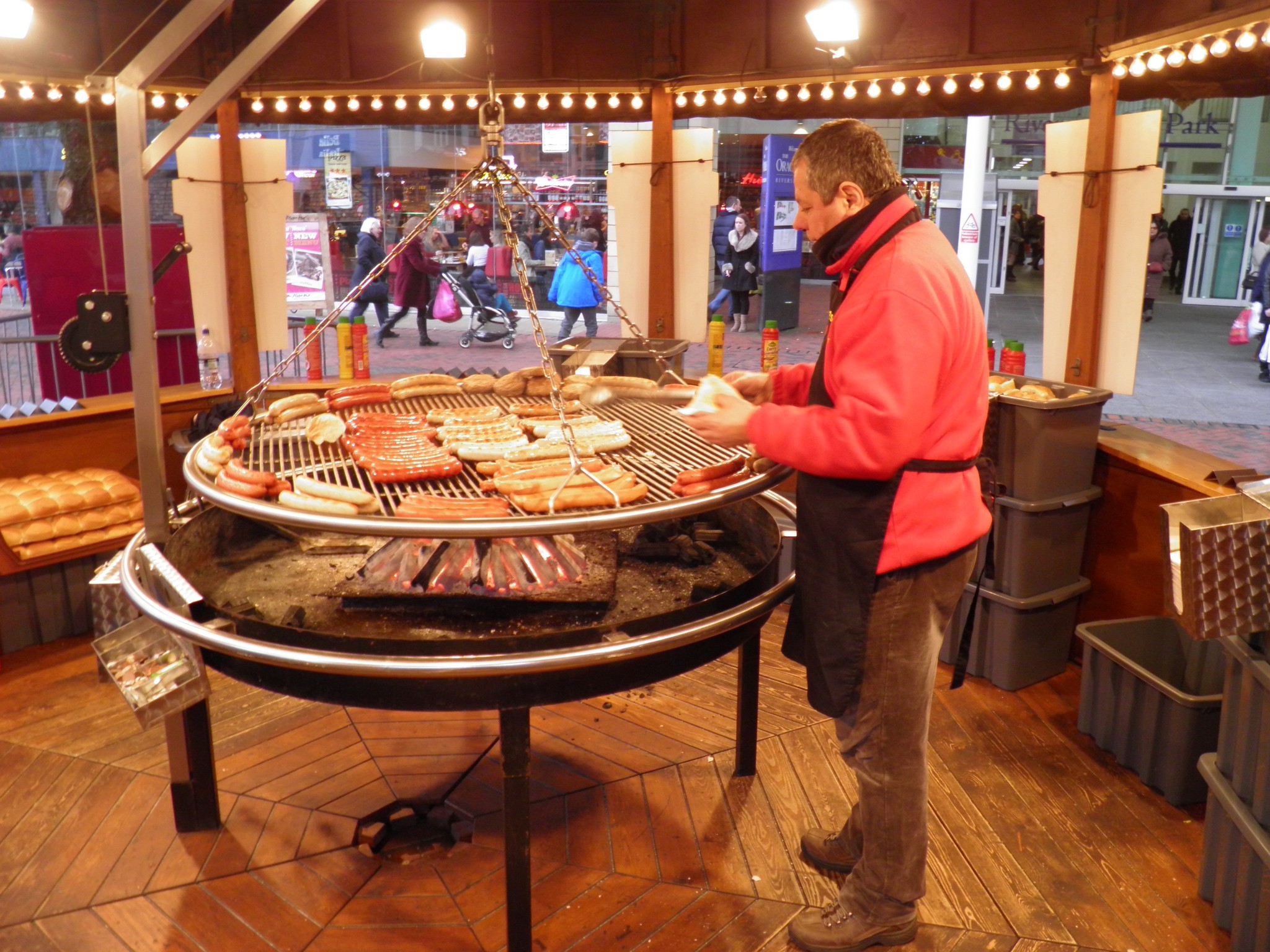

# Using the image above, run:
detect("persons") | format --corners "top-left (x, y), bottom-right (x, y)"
top-left (348, 216), bottom-right (401, 337)
top-left (706, 196), bottom-right (759, 332)
top-left (0, 221), bottom-right (32, 276)
top-left (372, 217), bottom-right (439, 347)
top-left (1142, 203), bottom-right (1203, 322)
top-left (469, 269), bottom-right (521, 323)
top-left (1246, 225), bottom-right (1270, 385)
top-left (997, 205), bottom-right (1045, 282)
top-left (548, 228), bottom-right (604, 342)
top-left (679, 118), bottom-right (994, 952)
top-left (385, 202), bottom-right (608, 279)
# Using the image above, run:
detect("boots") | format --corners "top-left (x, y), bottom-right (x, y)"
top-left (372, 314), bottom-right (397, 348)
top-left (1009, 265), bottom-right (1016, 278)
top-left (512, 310), bottom-right (518, 315)
top-left (507, 311), bottom-right (520, 322)
top-left (1006, 265), bottom-right (1010, 281)
top-left (416, 317), bottom-right (439, 346)
top-left (730, 313), bottom-right (741, 332)
top-left (738, 314), bottom-right (749, 333)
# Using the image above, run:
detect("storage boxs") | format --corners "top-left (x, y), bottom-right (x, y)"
top-left (548, 336), bottom-right (689, 382)
top-left (937, 370), bottom-right (1270, 952)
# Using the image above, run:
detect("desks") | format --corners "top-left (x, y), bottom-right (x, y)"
top-left (431, 256), bottom-right (466, 271)
top-left (534, 265), bottom-right (556, 270)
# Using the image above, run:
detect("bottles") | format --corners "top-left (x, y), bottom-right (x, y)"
top-left (197, 329), bottom-right (222, 390)
top-left (351, 315), bottom-right (369, 379)
top-left (1000, 339), bottom-right (1026, 377)
top-left (760, 320), bottom-right (779, 372)
top-left (988, 338), bottom-right (995, 372)
top-left (336, 316), bottom-right (355, 379)
top-left (706, 314), bottom-right (725, 379)
top-left (302, 316), bottom-right (323, 380)
top-left (466, 186), bottom-right (607, 204)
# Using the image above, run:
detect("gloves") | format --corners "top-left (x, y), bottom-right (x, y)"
top-left (440, 266), bottom-right (448, 273)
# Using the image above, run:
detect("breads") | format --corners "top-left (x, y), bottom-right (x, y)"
top-left (679, 374), bottom-right (742, 415)
top-left (988, 375), bottom-right (1089, 401)
top-left (303, 413), bottom-right (346, 445)
top-left (0, 466), bottom-right (146, 560)
top-left (460, 366), bottom-right (596, 400)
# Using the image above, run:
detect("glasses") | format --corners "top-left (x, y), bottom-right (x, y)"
top-left (1151, 226), bottom-right (1159, 230)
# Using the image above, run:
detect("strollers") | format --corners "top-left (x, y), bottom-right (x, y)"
top-left (442, 272), bottom-right (517, 349)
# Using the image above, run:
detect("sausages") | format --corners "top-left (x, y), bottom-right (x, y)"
top-left (195, 414), bottom-right (293, 498)
top-left (279, 475), bottom-right (381, 516)
top-left (671, 453), bottom-right (777, 496)
top-left (264, 373), bottom-right (461, 424)
top-left (342, 400), bottom-right (648, 519)
top-left (591, 375), bottom-right (698, 406)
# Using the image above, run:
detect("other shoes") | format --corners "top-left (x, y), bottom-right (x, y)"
top-left (1144, 309), bottom-right (1153, 322)
top-left (384, 331), bottom-right (400, 337)
top-left (1027, 259), bottom-right (1037, 266)
top-left (728, 316), bottom-right (734, 320)
top-left (1033, 261), bottom-right (1039, 270)
top-left (707, 306), bottom-right (715, 323)
top-left (1258, 370), bottom-right (1270, 382)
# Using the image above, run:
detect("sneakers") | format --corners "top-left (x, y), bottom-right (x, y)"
top-left (800, 828), bottom-right (858, 872)
top-left (787, 897), bottom-right (917, 952)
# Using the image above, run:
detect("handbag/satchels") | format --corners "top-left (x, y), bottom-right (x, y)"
top-left (1228, 307), bottom-right (1252, 345)
top-left (358, 275), bottom-right (388, 302)
top-left (1240, 272), bottom-right (1259, 289)
top-left (1248, 302), bottom-right (1265, 339)
top-left (433, 279), bottom-right (463, 323)
